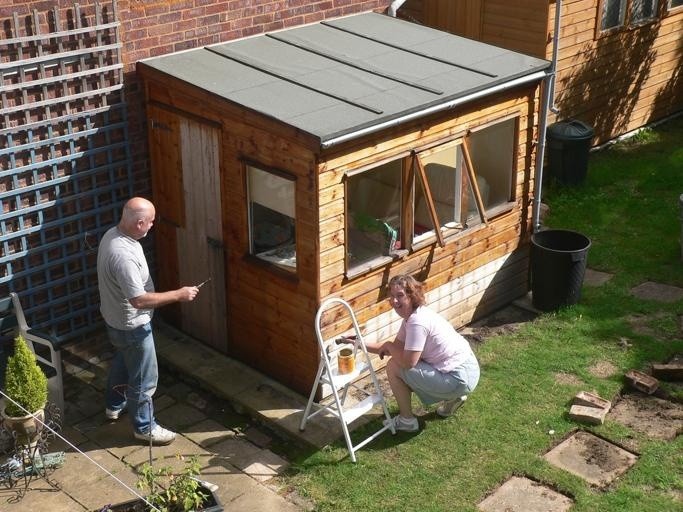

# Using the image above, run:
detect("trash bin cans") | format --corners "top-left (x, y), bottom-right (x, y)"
top-left (546, 118), bottom-right (596, 187)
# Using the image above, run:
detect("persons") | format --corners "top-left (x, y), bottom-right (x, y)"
top-left (96, 196), bottom-right (199, 447)
top-left (339, 275), bottom-right (481, 433)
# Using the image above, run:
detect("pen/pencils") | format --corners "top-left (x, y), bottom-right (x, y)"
top-left (197, 279), bottom-right (213, 288)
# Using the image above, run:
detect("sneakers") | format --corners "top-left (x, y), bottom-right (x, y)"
top-left (437, 395), bottom-right (467, 418)
top-left (382, 413), bottom-right (419, 433)
top-left (105, 399), bottom-right (128, 420)
top-left (134, 424), bottom-right (176, 446)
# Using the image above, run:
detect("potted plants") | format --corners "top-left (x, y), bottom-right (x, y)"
top-left (89, 451), bottom-right (225, 512)
top-left (0, 335), bottom-right (48, 444)
